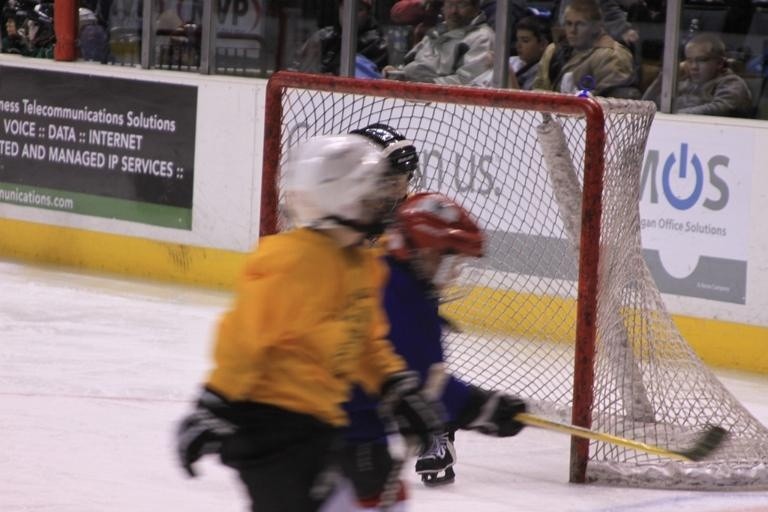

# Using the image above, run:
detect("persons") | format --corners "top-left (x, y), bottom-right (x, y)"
top-left (178, 122), bottom-right (528, 511)
top-left (0, 0), bottom-right (752, 115)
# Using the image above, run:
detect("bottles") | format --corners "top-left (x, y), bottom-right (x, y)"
top-left (688, 19), bottom-right (700, 39)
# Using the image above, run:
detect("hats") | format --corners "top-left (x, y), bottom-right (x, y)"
top-left (391, 1), bottom-right (439, 27)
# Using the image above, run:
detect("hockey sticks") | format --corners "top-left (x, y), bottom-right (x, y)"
top-left (514, 414), bottom-right (728, 463)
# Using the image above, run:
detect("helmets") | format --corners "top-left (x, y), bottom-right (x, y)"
top-left (386, 192), bottom-right (484, 288)
top-left (285, 134), bottom-right (391, 229)
top-left (1, 0), bottom-right (97, 48)
top-left (348, 122), bottom-right (419, 215)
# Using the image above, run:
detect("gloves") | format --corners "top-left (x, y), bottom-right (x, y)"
top-left (178, 387), bottom-right (241, 477)
top-left (375, 369), bottom-right (442, 446)
top-left (459, 384), bottom-right (527, 436)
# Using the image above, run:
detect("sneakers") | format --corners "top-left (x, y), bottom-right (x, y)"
top-left (416, 430), bottom-right (457, 474)
